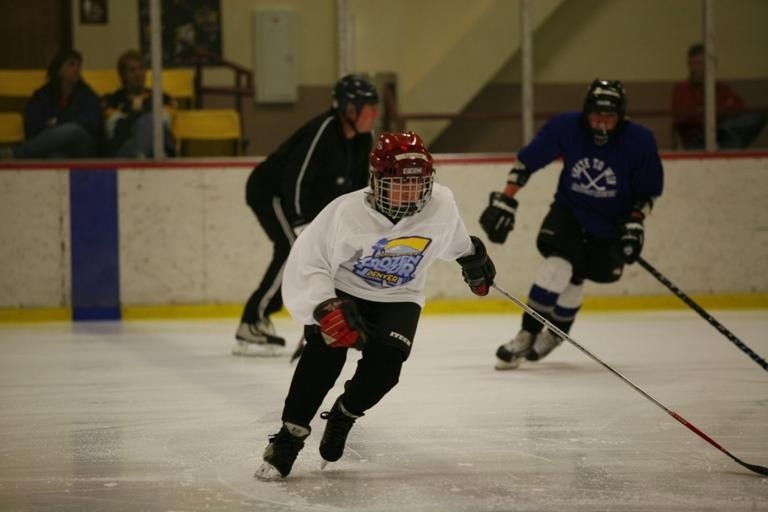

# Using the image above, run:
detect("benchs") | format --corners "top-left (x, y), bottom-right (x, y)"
top-left (0, 62), bottom-right (249, 164)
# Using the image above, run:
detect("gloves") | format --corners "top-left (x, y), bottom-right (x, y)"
top-left (456, 235), bottom-right (496, 296)
top-left (313, 296), bottom-right (371, 352)
top-left (621, 209), bottom-right (646, 264)
top-left (479, 191), bottom-right (519, 244)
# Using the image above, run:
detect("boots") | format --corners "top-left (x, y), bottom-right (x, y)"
top-left (318, 380), bottom-right (365, 461)
top-left (263, 416), bottom-right (312, 479)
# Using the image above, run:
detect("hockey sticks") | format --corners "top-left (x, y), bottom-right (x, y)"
top-left (493, 282), bottom-right (768, 477)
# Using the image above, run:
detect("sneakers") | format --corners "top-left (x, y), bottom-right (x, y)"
top-left (497, 329), bottom-right (532, 363)
top-left (235, 321), bottom-right (285, 348)
top-left (525, 330), bottom-right (564, 362)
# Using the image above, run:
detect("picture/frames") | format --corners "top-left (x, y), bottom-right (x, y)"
top-left (78, 0), bottom-right (111, 27)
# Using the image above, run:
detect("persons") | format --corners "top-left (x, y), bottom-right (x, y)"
top-left (234, 67), bottom-right (381, 345)
top-left (669, 43), bottom-right (767, 150)
top-left (480, 77), bottom-right (664, 362)
top-left (265, 129), bottom-right (498, 477)
top-left (101, 51), bottom-right (181, 160)
top-left (1, 49), bottom-right (102, 158)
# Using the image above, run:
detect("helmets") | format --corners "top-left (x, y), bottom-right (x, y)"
top-left (367, 131), bottom-right (436, 220)
top-left (584, 77), bottom-right (625, 147)
top-left (331, 73), bottom-right (379, 123)
